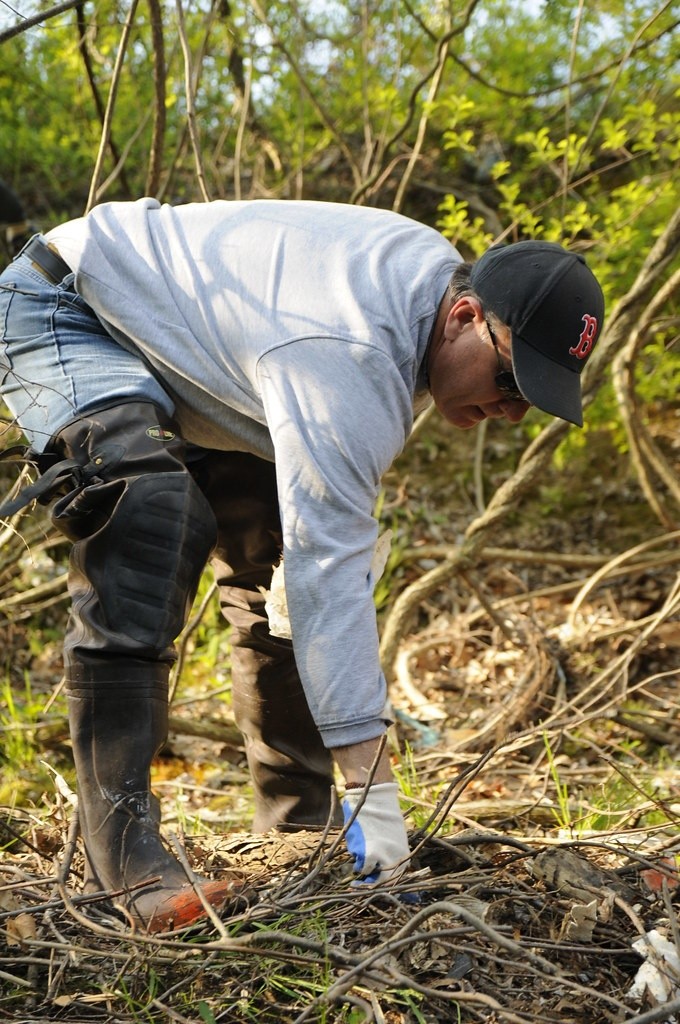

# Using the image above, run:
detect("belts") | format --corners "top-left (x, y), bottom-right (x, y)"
top-left (24, 237), bottom-right (76, 294)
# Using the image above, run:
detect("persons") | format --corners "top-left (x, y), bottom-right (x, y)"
top-left (0, 196), bottom-right (605, 933)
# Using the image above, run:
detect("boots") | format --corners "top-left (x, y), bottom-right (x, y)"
top-left (66, 660), bottom-right (244, 933)
top-left (232, 628), bottom-right (343, 831)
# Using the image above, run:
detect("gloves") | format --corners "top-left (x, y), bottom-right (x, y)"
top-left (338, 783), bottom-right (409, 890)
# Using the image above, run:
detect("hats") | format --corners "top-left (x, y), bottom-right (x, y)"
top-left (471, 242), bottom-right (606, 428)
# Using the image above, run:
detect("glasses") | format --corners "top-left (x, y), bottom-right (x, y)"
top-left (485, 319), bottom-right (529, 399)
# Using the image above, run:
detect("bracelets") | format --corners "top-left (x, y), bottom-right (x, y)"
top-left (345, 783), bottom-right (365, 789)
